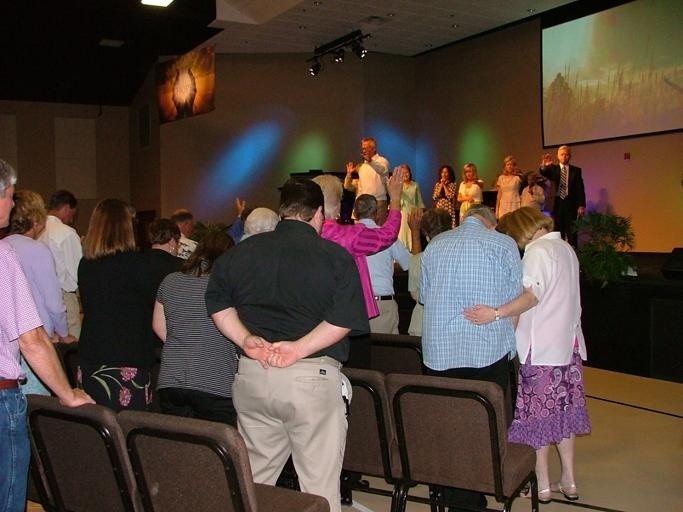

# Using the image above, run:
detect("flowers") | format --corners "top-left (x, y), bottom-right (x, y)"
top-left (75, 363), bottom-right (152, 407)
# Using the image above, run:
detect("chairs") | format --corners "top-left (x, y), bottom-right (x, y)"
top-left (24, 394), bottom-right (137, 512)
top-left (397, 372), bottom-right (540, 512)
top-left (337, 368), bottom-right (400, 511)
top-left (370, 332), bottom-right (424, 374)
top-left (117, 410), bottom-right (332, 512)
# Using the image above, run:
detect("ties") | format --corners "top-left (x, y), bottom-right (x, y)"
top-left (558, 165), bottom-right (570, 202)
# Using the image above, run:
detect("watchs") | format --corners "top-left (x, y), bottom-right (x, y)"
top-left (493, 307), bottom-right (501, 321)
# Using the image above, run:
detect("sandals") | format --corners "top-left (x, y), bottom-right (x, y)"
top-left (517, 480), bottom-right (552, 504)
top-left (550, 478), bottom-right (580, 501)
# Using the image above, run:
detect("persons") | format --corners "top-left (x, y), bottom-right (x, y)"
top-left (150, 228), bottom-right (239, 426)
top-left (311, 165), bottom-right (408, 364)
top-left (458, 162), bottom-right (485, 224)
top-left (430, 164), bottom-right (458, 230)
top-left (417, 207), bottom-right (523, 512)
top-left (223, 191), bottom-right (279, 245)
top-left (35, 189), bottom-right (84, 344)
top-left (142, 217), bottom-right (184, 281)
top-left (539, 144), bottom-right (587, 249)
top-left (392, 163), bottom-right (428, 264)
top-left (0, 188), bottom-right (78, 398)
top-left (170, 207), bottom-right (202, 261)
top-left (74, 195), bottom-right (162, 410)
top-left (342, 159), bottom-right (362, 222)
top-left (351, 192), bottom-right (415, 335)
top-left (458, 204), bottom-right (592, 503)
top-left (204, 176), bottom-right (371, 512)
top-left (408, 209), bottom-right (456, 338)
top-left (521, 171), bottom-right (547, 212)
top-left (494, 154), bottom-right (525, 223)
top-left (0, 158), bottom-right (99, 512)
top-left (354, 137), bottom-right (391, 226)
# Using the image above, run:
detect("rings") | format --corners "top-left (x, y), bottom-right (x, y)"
top-left (399, 182), bottom-right (403, 184)
top-left (472, 318), bottom-right (478, 325)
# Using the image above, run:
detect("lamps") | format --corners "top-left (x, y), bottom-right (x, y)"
top-left (306, 29), bottom-right (373, 80)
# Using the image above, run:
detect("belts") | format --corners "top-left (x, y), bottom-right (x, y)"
top-left (0, 377), bottom-right (22, 388)
